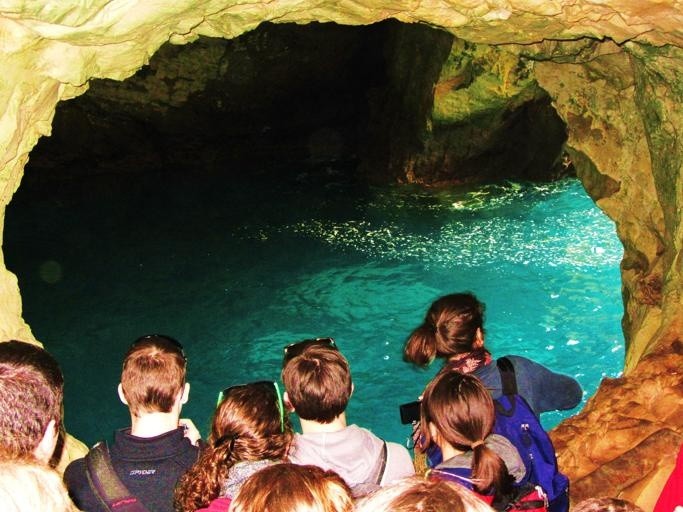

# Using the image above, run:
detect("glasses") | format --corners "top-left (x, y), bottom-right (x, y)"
top-left (283, 336), bottom-right (340, 365)
top-left (217, 380), bottom-right (285, 438)
top-left (131, 334), bottom-right (183, 351)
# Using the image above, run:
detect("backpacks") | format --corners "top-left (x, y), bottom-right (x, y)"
top-left (432, 474), bottom-right (549, 512)
top-left (428, 356), bottom-right (571, 512)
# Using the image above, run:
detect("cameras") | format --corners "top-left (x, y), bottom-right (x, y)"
top-left (399, 400), bottom-right (424, 424)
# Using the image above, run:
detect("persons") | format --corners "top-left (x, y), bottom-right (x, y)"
top-left (410, 371), bottom-right (526, 504)
top-left (0, 451), bottom-right (78, 512)
top-left (401, 289), bottom-right (582, 424)
top-left (170, 378), bottom-right (296, 512)
top-left (0, 337), bottom-right (67, 467)
top-left (61, 332), bottom-right (209, 512)
top-left (358, 477), bottom-right (496, 512)
top-left (569, 495), bottom-right (644, 511)
top-left (226, 463), bottom-right (356, 512)
top-left (278, 336), bottom-right (415, 494)
